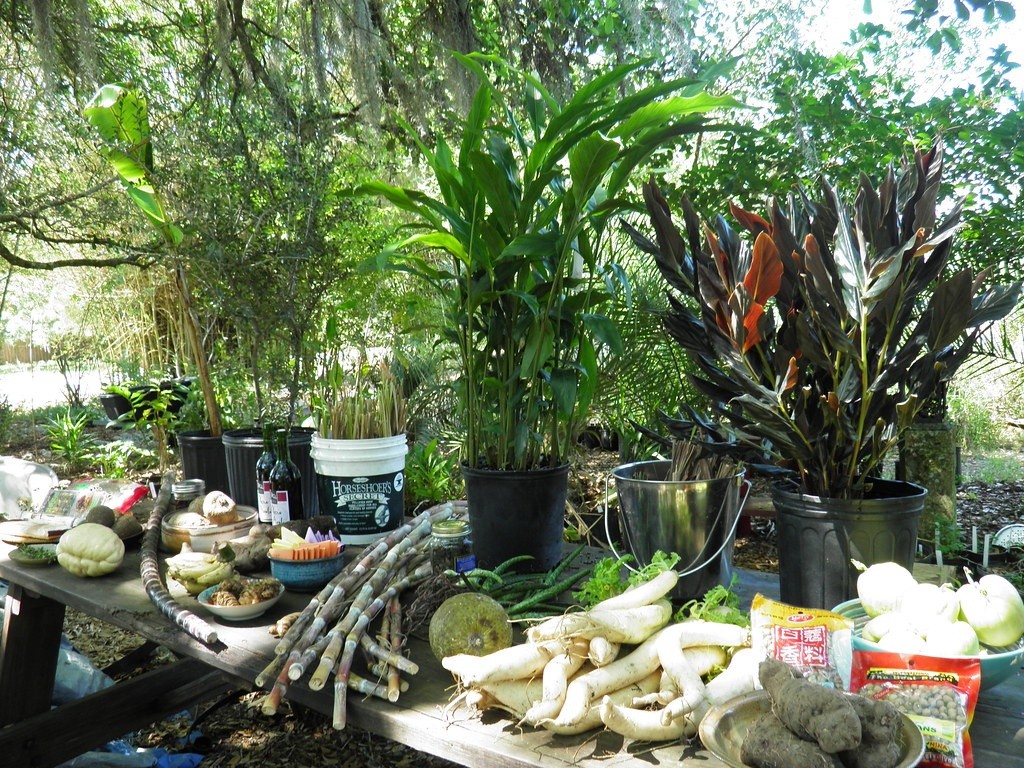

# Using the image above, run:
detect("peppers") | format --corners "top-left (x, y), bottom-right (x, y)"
top-left (857, 562), bottom-right (1024, 660)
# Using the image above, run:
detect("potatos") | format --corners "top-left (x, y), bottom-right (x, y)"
top-left (740, 658), bottom-right (904, 768)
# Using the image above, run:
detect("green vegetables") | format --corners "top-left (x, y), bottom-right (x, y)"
top-left (18, 545), bottom-right (55, 563)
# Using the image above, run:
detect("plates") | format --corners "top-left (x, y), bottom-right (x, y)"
top-left (8, 543), bottom-right (57, 568)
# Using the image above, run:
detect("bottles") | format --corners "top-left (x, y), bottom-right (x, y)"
top-left (270, 429), bottom-right (304, 526)
top-left (431, 521), bottom-right (476, 578)
top-left (171, 478), bottom-right (206, 509)
top-left (255, 426), bottom-right (280, 525)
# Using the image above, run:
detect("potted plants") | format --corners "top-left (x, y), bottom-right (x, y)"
top-left (613, 131), bottom-right (1024, 611)
top-left (309, 362), bottom-right (408, 546)
top-left (222, 239), bottom-right (321, 523)
top-left (81, 82), bottom-right (235, 499)
top-left (99, 353), bottom-right (193, 422)
top-left (333, 46), bottom-right (763, 578)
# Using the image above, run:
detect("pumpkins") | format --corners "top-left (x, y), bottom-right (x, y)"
top-left (56, 522), bottom-right (124, 577)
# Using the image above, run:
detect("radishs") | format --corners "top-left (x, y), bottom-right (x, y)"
top-left (442, 553), bottom-right (753, 741)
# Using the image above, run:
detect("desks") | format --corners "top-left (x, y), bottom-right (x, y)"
top-left (0, 477), bottom-right (1024, 768)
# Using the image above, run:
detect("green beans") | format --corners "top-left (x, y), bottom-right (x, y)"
top-left (861, 685), bottom-right (965, 725)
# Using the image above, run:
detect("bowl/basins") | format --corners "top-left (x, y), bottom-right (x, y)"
top-left (161, 505), bottom-right (258, 553)
top-left (268, 545), bottom-right (347, 584)
top-left (829, 596), bottom-right (1024, 696)
top-left (698, 689), bottom-right (926, 768)
top-left (197, 579), bottom-right (285, 620)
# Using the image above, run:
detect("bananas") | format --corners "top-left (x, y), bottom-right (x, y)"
top-left (167, 556), bottom-right (234, 595)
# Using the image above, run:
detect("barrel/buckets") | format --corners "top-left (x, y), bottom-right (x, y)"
top-left (604, 460), bottom-right (753, 613)
top-left (310, 430), bottom-right (409, 546)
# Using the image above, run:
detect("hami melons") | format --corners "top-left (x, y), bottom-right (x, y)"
top-left (429, 594), bottom-right (513, 662)
